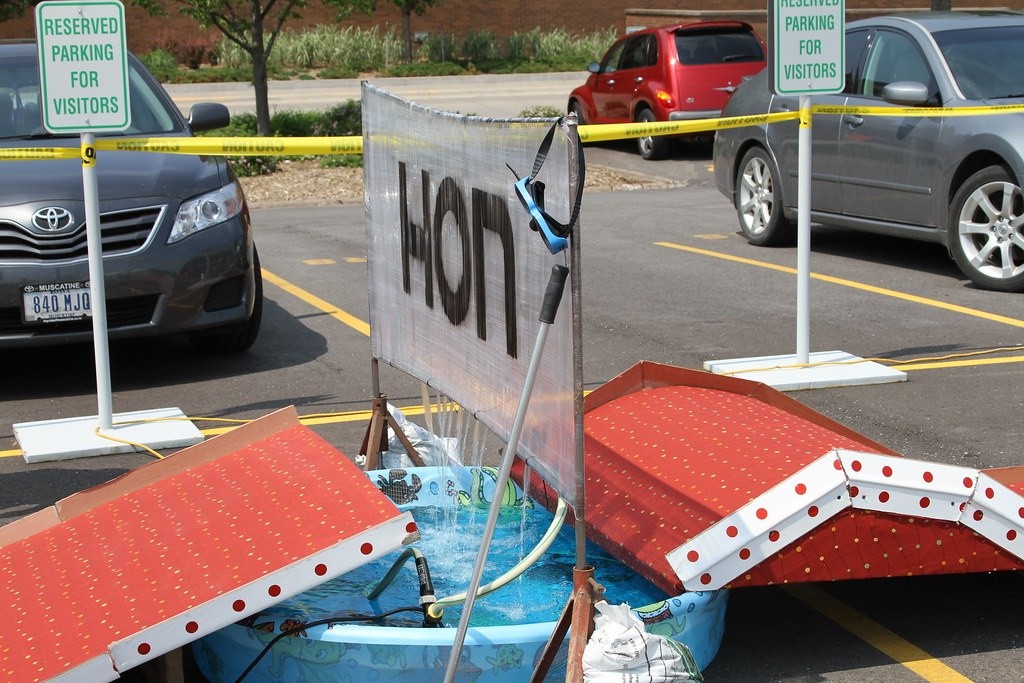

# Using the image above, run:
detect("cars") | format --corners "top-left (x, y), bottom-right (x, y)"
top-left (567, 19), bottom-right (768, 160)
top-left (712, 7), bottom-right (1024, 293)
top-left (0, 41), bottom-right (264, 355)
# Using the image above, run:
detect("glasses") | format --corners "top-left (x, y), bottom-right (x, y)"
top-left (514, 116), bottom-right (586, 255)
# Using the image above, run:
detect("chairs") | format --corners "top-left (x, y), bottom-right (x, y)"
top-left (894, 49), bottom-right (931, 85)
top-left (694, 45), bottom-right (716, 58)
top-left (0, 93), bottom-right (15, 134)
top-left (679, 49), bottom-right (692, 65)
